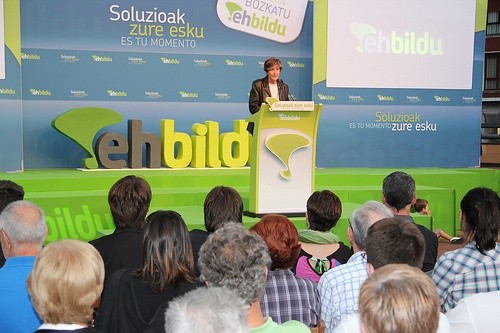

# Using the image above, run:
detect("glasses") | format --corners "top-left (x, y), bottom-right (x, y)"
top-left (146, 210), bottom-right (182, 224)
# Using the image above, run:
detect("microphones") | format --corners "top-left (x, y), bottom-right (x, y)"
top-left (281, 79), bottom-right (294, 101)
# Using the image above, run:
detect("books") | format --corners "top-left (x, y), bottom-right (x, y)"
top-left (482, 11), bottom-right (500, 138)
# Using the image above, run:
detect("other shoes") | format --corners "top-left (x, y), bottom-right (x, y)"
top-left (449, 238), bottom-right (464, 245)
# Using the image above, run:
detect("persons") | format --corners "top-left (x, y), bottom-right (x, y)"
top-left (246, 58), bottom-right (288, 134)
top-left (431, 187), bottom-right (500, 314)
top-left (334, 217), bottom-right (450, 333)
top-left (88, 175), bottom-right (151, 281)
top-left (189, 186), bottom-right (243, 261)
top-left (382, 171), bottom-right (437, 273)
top-left (318, 201), bottom-right (393, 333)
top-left (95, 210), bottom-right (202, 333)
top-left (165, 286), bottom-right (249, 333)
top-left (446, 291), bottom-right (500, 333)
top-left (414, 199), bottom-right (464, 243)
top-left (27, 240), bottom-right (106, 333)
top-left (198, 222), bottom-right (312, 333)
top-left (291, 190), bottom-right (353, 283)
top-left (358, 263), bottom-right (440, 333)
top-left (0, 180), bottom-right (24, 268)
top-left (0, 200), bottom-right (48, 333)
top-left (249, 214), bottom-right (320, 328)
